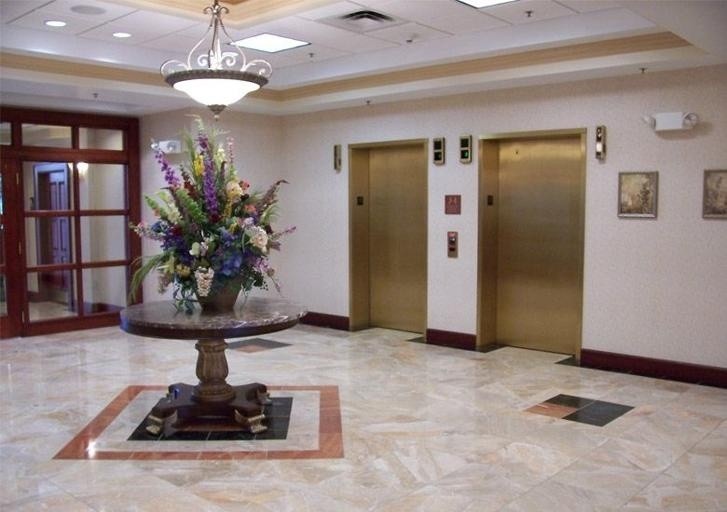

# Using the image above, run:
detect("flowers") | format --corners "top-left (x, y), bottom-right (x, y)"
top-left (125, 113), bottom-right (296, 314)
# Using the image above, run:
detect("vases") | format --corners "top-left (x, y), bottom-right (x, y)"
top-left (192, 282), bottom-right (240, 312)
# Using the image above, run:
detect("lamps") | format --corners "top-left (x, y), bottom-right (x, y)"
top-left (158, 0), bottom-right (272, 120)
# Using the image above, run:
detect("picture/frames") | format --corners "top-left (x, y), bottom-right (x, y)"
top-left (702, 167), bottom-right (727, 217)
top-left (616, 170), bottom-right (661, 216)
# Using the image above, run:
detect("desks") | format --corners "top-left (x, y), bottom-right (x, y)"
top-left (120, 299), bottom-right (309, 434)
